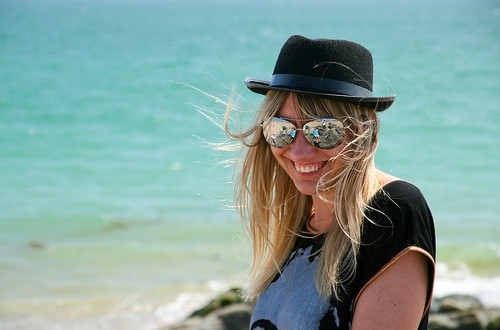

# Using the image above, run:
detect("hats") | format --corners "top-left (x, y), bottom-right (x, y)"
top-left (246, 34), bottom-right (396, 113)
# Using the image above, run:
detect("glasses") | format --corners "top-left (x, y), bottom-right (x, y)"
top-left (260, 117), bottom-right (352, 148)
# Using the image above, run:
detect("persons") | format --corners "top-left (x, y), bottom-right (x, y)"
top-left (184, 35), bottom-right (436, 330)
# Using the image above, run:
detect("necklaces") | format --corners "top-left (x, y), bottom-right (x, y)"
top-left (306, 208), bottom-right (319, 233)
